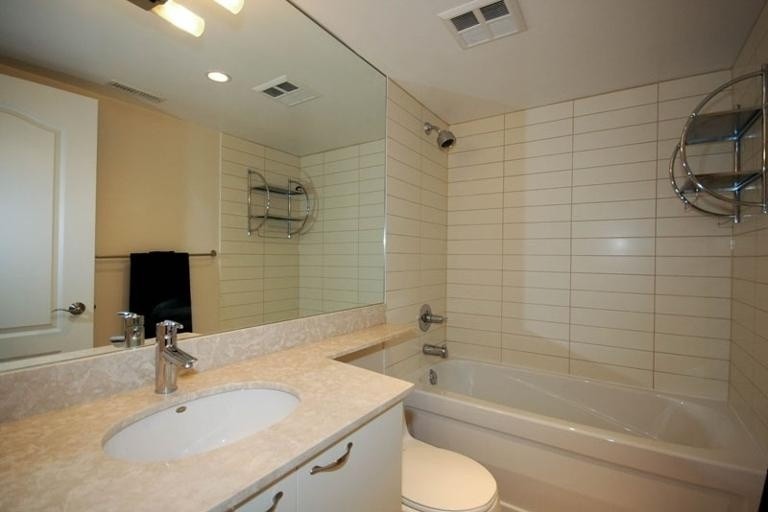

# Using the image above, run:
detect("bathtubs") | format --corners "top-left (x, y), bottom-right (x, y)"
top-left (401, 357), bottom-right (764, 494)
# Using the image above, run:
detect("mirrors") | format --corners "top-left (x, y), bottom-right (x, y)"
top-left (0, 0), bottom-right (385, 373)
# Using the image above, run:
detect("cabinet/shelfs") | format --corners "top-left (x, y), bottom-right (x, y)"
top-left (665, 64), bottom-right (765, 224)
top-left (232, 398), bottom-right (404, 510)
top-left (244, 163), bottom-right (310, 237)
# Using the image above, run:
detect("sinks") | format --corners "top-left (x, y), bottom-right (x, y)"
top-left (101, 381), bottom-right (303, 464)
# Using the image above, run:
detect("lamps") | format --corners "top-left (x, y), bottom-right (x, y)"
top-left (140, 0), bottom-right (245, 37)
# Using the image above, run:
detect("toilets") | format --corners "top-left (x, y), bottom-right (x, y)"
top-left (401, 404), bottom-right (498, 512)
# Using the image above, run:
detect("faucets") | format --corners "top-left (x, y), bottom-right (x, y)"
top-left (424, 344), bottom-right (449, 359)
top-left (155, 319), bottom-right (199, 394)
top-left (110, 311), bottom-right (145, 348)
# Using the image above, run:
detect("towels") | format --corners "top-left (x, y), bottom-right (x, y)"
top-left (127, 249), bottom-right (195, 340)
